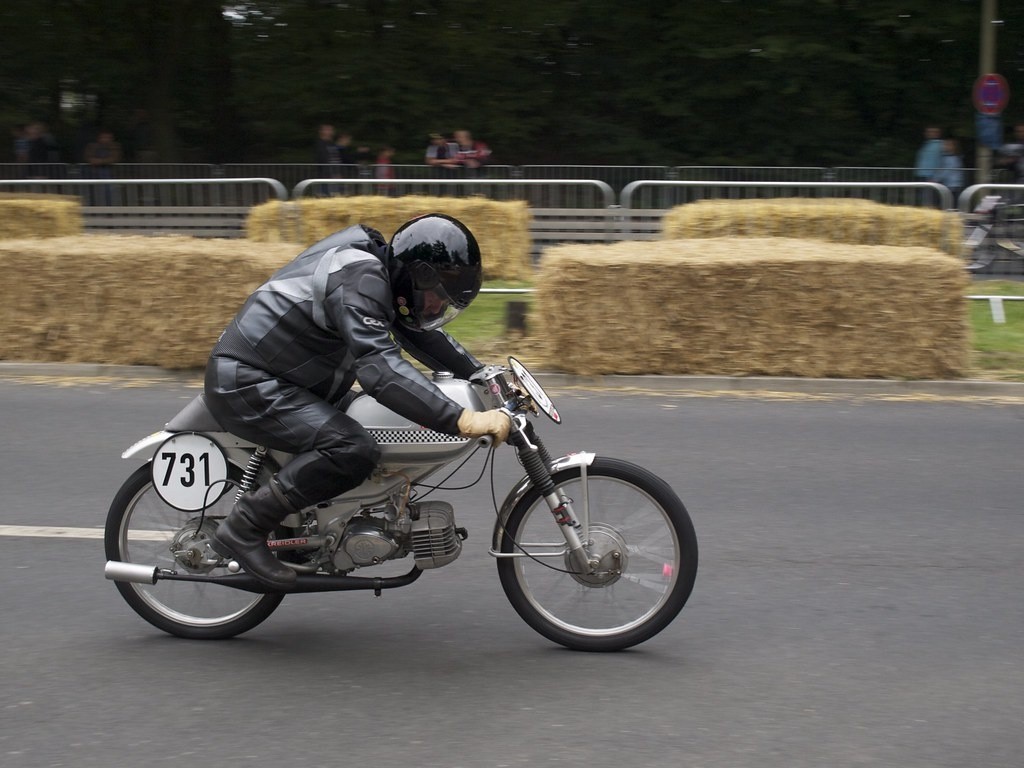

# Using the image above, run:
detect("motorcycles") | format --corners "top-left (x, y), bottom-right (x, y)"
top-left (103, 357), bottom-right (698, 650)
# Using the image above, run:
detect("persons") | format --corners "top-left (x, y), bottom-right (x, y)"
top-left (911, 118), bottom-right (1024, 214)
top-left (314, 121), bottom-right (500, 201)
top-left (202, 211), bottom-right (533, 587)
top-left (13, 118), bottom-right (130, 205)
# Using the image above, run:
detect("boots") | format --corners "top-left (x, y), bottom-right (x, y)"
top-left (210, 477), bottom-right (299, 588)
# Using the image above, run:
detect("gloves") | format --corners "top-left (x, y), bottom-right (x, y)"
top-left (457, 408), bottom-right (511, 450)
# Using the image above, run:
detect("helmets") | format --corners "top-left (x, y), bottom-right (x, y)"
top-left (386, 213), bottom-right (483, 333)
